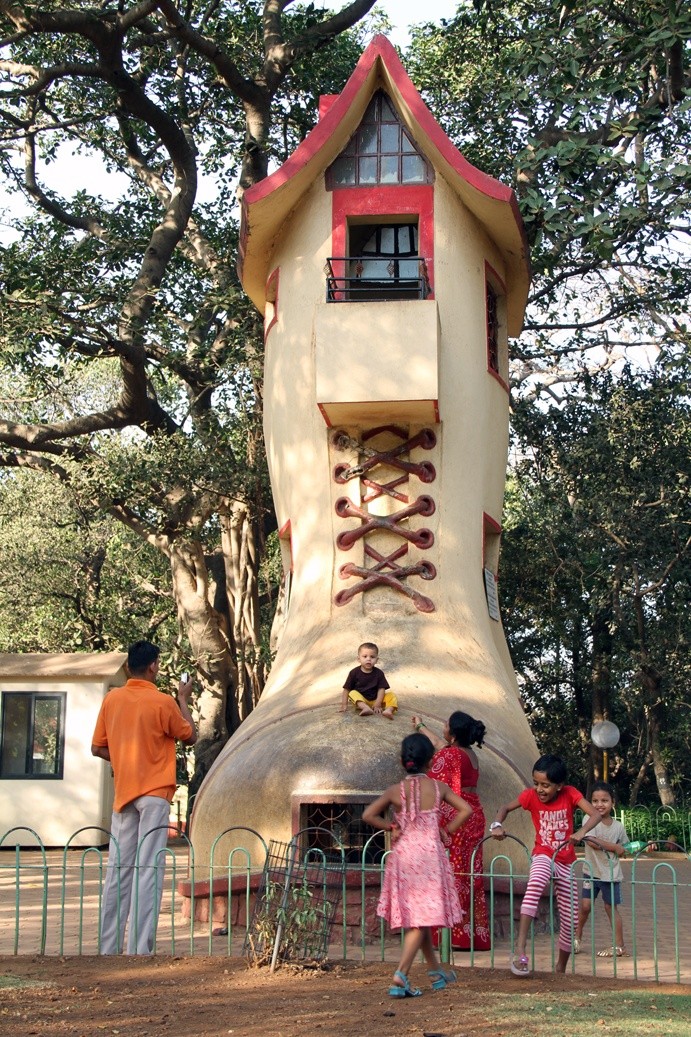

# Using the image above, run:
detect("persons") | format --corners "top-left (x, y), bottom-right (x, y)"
top-left (574, 782), bottom-right (656, 957)
top-left (490, 756), bottom-right (602, 975)
top-left (91, 640), bottom-right (197, 955)
top-left (362, 733), bottom-right (473, 996)
top-left (337, 643), bottom-right (398, 720)
top-left (411, 711), bottom-right (491, 950)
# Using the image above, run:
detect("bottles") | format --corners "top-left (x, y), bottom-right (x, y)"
top-left (616, 841), bottom-right (657, 855)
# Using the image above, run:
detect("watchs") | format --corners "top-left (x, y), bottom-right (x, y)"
top-left (490, 822), bottom-right (502, 829)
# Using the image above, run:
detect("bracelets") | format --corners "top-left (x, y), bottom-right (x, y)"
top-left (389, 822), bottom-right (396, 831)
top-left (444, 827), bottom-right (452, 837)
top-left (415, 723), bottom-right (425, 731)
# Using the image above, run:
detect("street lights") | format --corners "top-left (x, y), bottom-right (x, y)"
top-left (591, 721), bottom-right (620, 784)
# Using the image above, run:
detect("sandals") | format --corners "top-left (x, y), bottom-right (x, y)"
top-left (596, 946), bottom-right (626, 958)
top-left (387, 969), bottom-right (425, 998)
top-left (428, 967), bottom-right (460, 989)
top-left (508, 953), bottom-right (532, 976)
top-left (573, 939), bottom-right (581, 955)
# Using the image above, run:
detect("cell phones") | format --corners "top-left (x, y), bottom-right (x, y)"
top-left (181, 672), bottom-right (188, 685)
